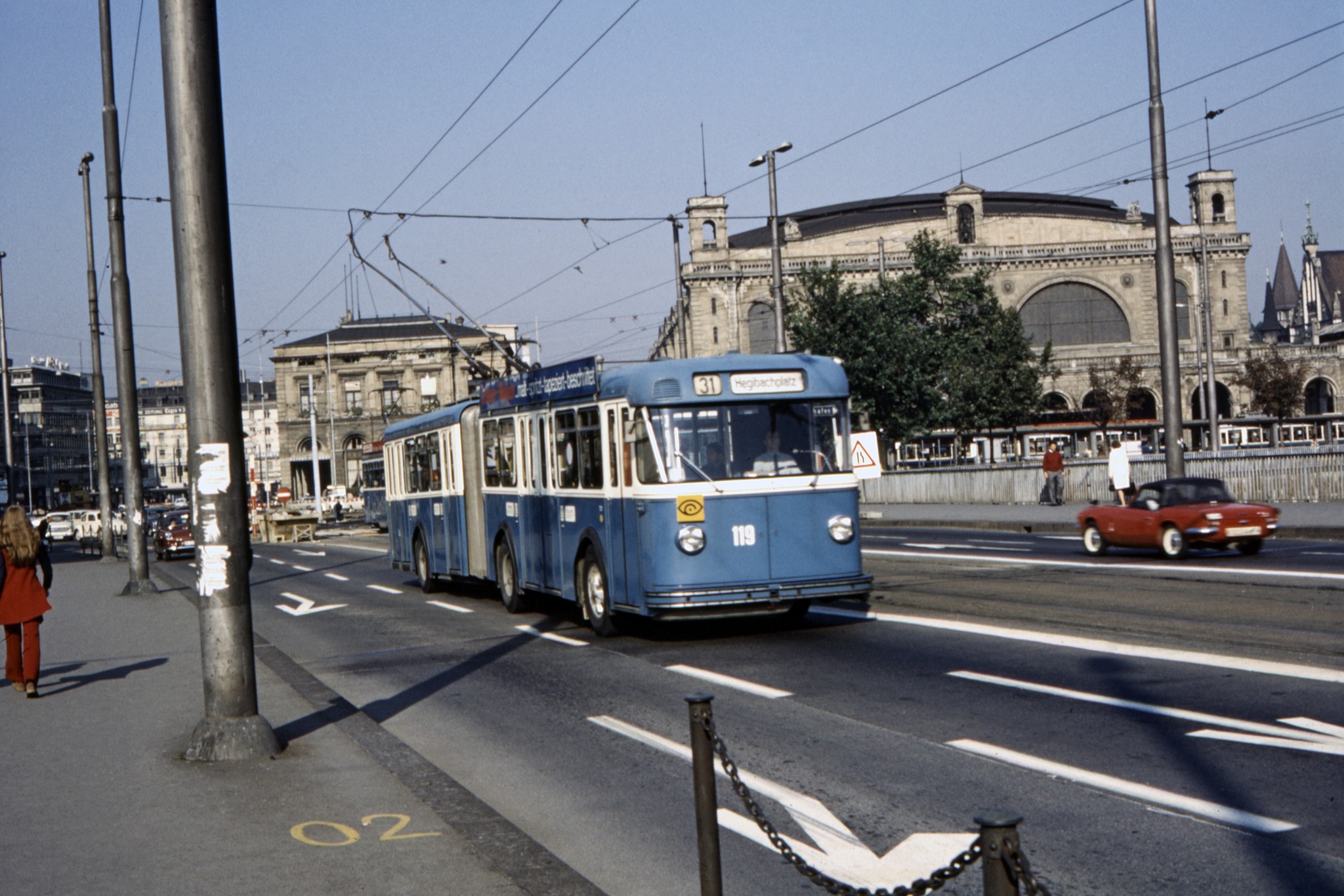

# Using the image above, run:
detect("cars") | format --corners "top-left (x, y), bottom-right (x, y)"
top-left (27, 498), bottom-right (194, 544)
top-left (153, 508), bottom-right (196, 561)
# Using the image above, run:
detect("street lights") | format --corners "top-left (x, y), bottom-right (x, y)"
top-left (85, 410), bottom-right (93, 493)
top-left (1191, 302), bottom-right (1209, 449)
top-left (76, 150), bottom-right (118, 566)
top-left (24, 408), bottom-right (32, 512)
top-left (747, 140), bottom-right (795, 354)
top-left (0, 251), bottom-right (16, 507)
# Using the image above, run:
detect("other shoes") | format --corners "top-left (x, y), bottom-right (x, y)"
top-left (11, 681), bottom-right (38, 695)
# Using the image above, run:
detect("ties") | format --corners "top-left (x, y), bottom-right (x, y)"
top-left (773, 455), bottom-right (779, 475)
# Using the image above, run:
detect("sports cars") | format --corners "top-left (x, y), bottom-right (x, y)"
top-left (1079, 477), bottom-right (1280, 556)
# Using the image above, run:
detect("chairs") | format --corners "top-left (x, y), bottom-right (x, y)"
top-left (1165, 491), bottom-right (1219, 504)
top-left (486, 450), bottom-right (762, 488)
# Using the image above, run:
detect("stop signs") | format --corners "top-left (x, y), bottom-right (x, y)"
top-left (278, 487), bottom-right (293, 503)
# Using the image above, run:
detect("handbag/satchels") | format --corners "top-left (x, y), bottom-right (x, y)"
top-left (1124, 478), bottom-right (1136, 495)
top-left (1109, 480), bottom-right (1115, 491)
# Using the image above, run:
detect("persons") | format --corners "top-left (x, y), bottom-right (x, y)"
top-left (753, 427), bottom-right (803, 476)
top-left (1, 506), bottom-right (53, 698)
top-left (1108, 440), bottom-right (1131, 506)
top-left (33, 519), bottom-right (53, 599)
top-left (1043, 441), bottom-right (1065, 506)
top-left (168, 517), bottom-right (177, 528)
top-left (698, 441), bottom-right (741, 480)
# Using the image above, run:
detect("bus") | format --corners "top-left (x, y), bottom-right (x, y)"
top-left (360, 454), bottom-right (390, 535)
top-left (379, 352), bottom-right (874, 631)
